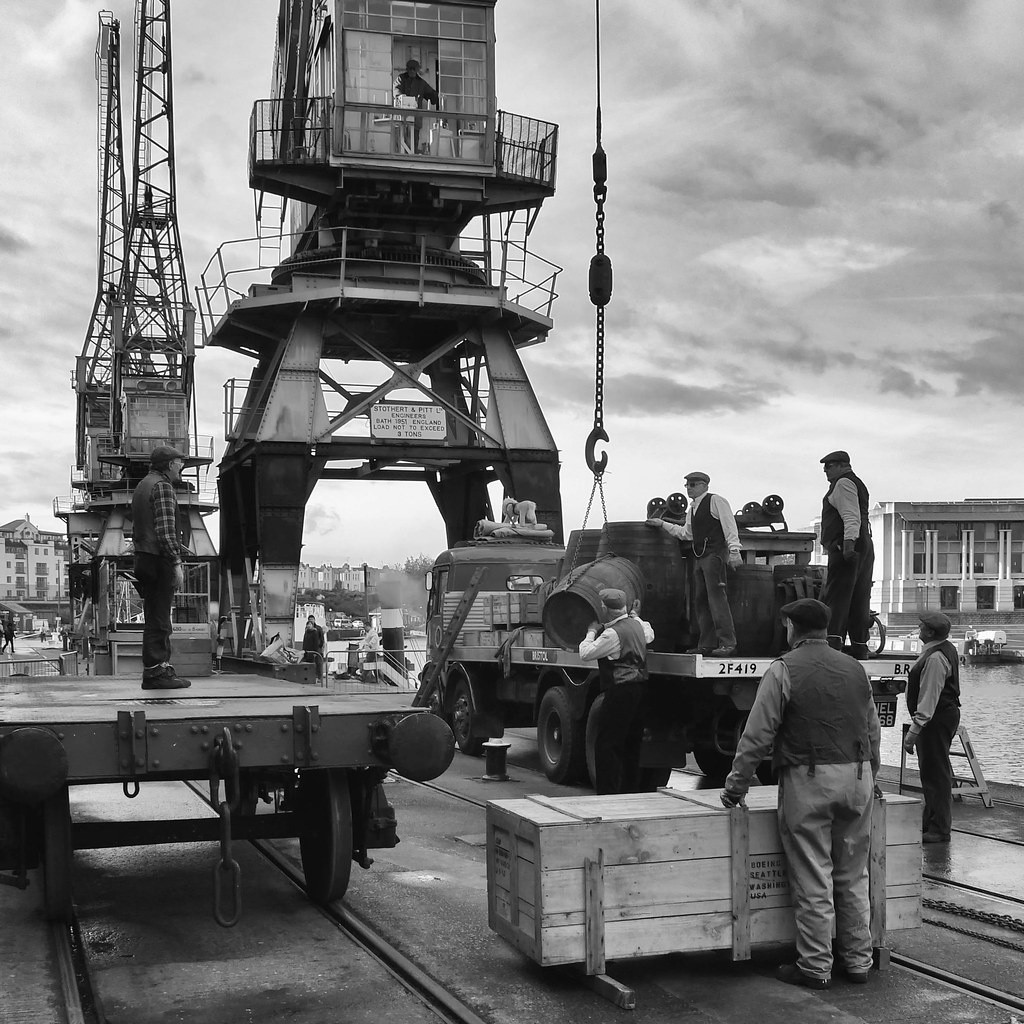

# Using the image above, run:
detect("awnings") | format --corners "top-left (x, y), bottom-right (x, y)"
top-left (0, 602), bottom-right (31, 613)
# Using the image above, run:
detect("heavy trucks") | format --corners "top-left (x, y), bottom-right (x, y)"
top-left (419, 497), bottom-right (921, 795)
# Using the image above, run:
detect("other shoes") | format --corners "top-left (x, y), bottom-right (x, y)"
top-left (685, 646), bottom-right (716, 656)
top-left (777, 964), bottom-right (830, 989)
top-left (827, 637), bottom-right (843, 650)
top-left (142, 664), bottom-right (191, 689)
top-left (842, 643), bottom-right (868, 659)
top-left (712, 645), bottom-right (737, 657)
top-left (845, 964), bottom-right (876, 983)
top-left (922, 832), bottom-right (951, 843)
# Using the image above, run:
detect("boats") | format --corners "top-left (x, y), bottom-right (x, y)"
top-left (960, 625), bottom-right (1024, 665)
top-left (898, 629), bottom-right (952, 641)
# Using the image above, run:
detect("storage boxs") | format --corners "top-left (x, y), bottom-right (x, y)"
top-left (485, 783), bottom-right (921, 968)
top-left (462, 592), bottom-right (551, 648)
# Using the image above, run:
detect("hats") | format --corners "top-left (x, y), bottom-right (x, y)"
top-left (780, 598), bottom-right (830, 630)
top-left (684, 472), bottom-right (710, 484)
top-left (820, 451), bottom-right (850, 463)
top-left (150, 446), bottom-right (186, 464)
top-left (918, 611), bottom-right (951, 640)
top-left (599, 589), bottom-right (627, 609)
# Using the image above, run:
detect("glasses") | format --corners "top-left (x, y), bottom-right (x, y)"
top-left (173, 462), bottom-right (184, 469)
top-left (825, 463), bottom-right (838, 469)
top-left (685, 482), bottom-right (706, 488)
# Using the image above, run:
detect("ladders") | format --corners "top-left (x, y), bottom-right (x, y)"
top-left (899, 722), bottom-right (994, 808)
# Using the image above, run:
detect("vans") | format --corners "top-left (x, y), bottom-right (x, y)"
top-left (352, 621), bottom-right (364, 628)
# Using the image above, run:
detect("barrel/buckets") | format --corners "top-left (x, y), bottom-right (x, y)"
top-left (726, 564), bottom-right (776, 658)
top-left (542, 557), bottom-right (647, 654)
top-left (773, 564), bottom-right (824, 656)
top-left (559, 529), bottom-right (602, 585)
top-left (597, 521), bottom-right (686, 649)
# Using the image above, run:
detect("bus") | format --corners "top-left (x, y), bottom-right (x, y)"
top-left (334, 619), bottom-right (352, 628)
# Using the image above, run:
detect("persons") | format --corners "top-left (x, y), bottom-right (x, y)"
top-left (0, 620), bottom-right (17, 654)
top-left (393, 59), bottom-right (440, 154)
top-left (357, 622), bottom-right (379, 679)
top-left (302, 615), bottom-right (324, 682)
top-left (904, 612), bottom-right (960, 842)
top-left (579, 588), bottom-right (655, 795)
top-left (820, 450), bottom-right (874, 659)
top-left (720, 598), bottom-right (881, 987)
top-left (132, 446), bottom-right (191, 690)
top-left (646, 472), bottom-right (743, 657)
top-left (40, 632), bottom-right (46, 642)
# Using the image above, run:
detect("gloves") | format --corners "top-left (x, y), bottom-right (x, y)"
top-left (720, 788), bottom-right (747, 808)
top-left (874, 785), bottom-right (883, 799)
top-left (632, 599), bottom-right (642, 616)
top-left (646, 518), bottom-right (663, 528)
top-left (727, 551), bottom-right (744, 572)
top-left (588, 620), bottom-right (604, 632)
top-left (903, 731), bottom-right (918, 755)
top-left (843, 540), bottom-right (858, 564)
